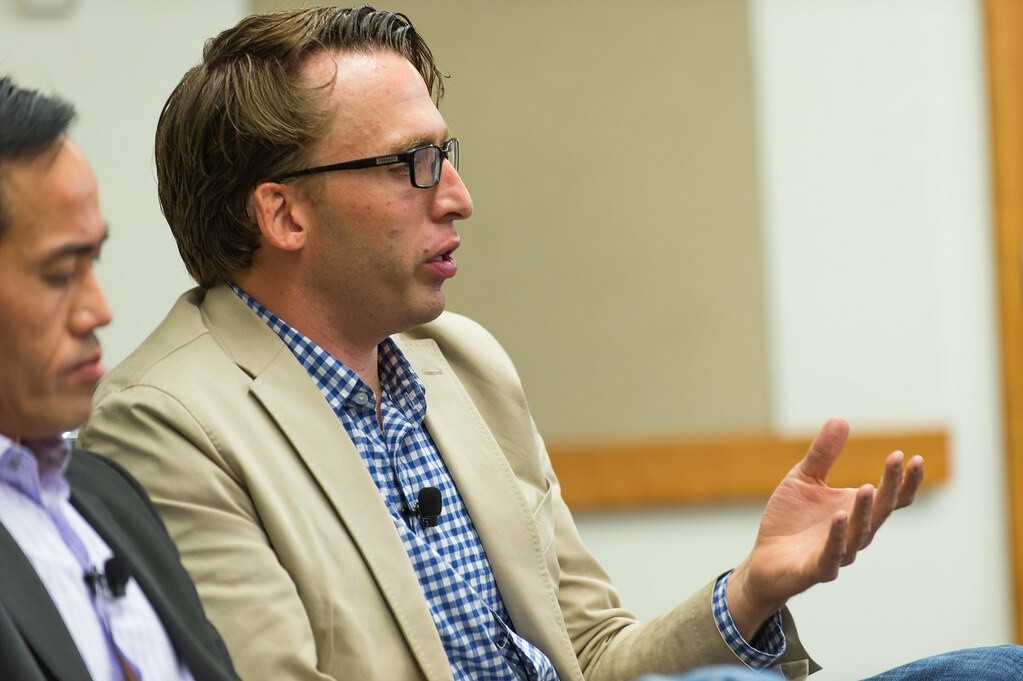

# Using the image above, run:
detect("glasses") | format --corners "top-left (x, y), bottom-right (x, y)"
top-left (264, 136), bottom-right (459, 191)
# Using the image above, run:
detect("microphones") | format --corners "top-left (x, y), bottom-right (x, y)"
top-left (417, 487), bottom-right (442, 527)
top-left (105, 559), bottom-right (130, 597)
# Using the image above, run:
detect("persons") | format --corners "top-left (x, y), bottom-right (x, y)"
top-left (74, 5), bottom-right (1023, 681)
top-left (0, 74), bottom-right (243, 681)
